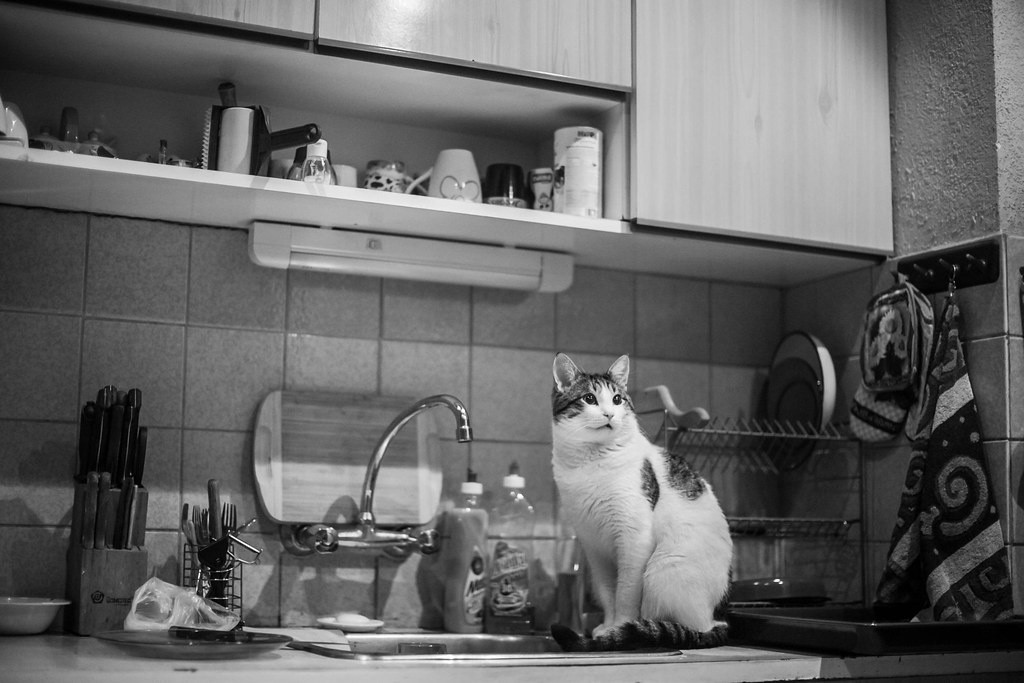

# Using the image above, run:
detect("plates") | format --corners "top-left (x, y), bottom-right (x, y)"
top-left (316, 617), bottom-right (384, 631)
top-left (770, 330), bottom-right (836, 434)
top-left (758, 358), bottom-right (823, 473)
top-left (731, 579), bottom-right (833, 602)
top-left (90, 628), bottom-right (293, 660)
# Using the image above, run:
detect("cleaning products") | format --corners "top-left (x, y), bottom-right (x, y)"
top-left (440, 458), bottom-right (540, 633)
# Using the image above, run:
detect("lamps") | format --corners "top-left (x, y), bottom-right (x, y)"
top-left (248, 221), bottom-right (575, 294)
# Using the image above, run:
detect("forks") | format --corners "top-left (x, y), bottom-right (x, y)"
top-left (192, 502), bottom-right (237, 608)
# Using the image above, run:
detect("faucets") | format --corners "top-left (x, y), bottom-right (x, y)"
top-left (307, 392), bottom-right (475, 555)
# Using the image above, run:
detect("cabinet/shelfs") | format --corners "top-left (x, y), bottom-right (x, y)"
top-left (654, 406), bottom-right (875, 612)
top-left (620, 0), bottom-right (895, 290)
top-left (0, 0), bottom-right (634, 143)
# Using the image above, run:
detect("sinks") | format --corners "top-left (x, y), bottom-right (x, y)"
top-left (289, 630), bottom-right (685, 662)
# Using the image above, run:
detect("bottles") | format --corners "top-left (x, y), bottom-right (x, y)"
top-left (486, 461), bottom-right (535, 635)
top-left (302, 139), bottom-right (330, 185)
top-left (556, 506), bottom-right (584, 634)
top-left (444, 469), bottom-right (488, 633)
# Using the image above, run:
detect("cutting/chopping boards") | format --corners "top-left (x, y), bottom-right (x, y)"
top-left (251, 390), bottom-right (442, 525)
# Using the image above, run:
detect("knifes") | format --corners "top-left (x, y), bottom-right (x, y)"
top-left (64, 386), bottom-right (148, 550)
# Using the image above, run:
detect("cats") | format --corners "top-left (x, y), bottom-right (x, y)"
top-left (549, 351), bottom-right (735, 651)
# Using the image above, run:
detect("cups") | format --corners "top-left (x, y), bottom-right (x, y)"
top-left (530, 167), bottom-right (553, 211)
top-left (404, 149), bottom-right (482, 203)
top-left (484, 163), bottom-right (530, 208)
top-left (364, 160), bottom-right (426, 196)
top-left (3, 101), bottom-right (29, 148)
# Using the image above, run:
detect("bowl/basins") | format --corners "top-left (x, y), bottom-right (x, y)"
top-left (0, 597), bottom-right (71, 635)
